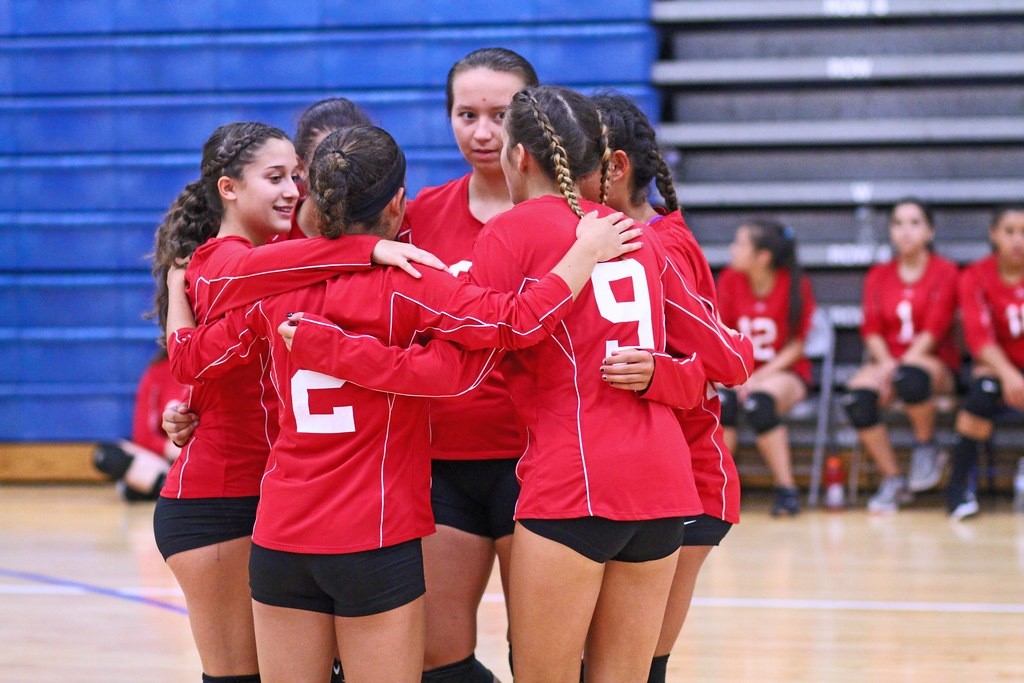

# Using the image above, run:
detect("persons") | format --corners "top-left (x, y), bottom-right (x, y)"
top-left (844, 199), bottom-right (965, 512)
top-left (90, 48), bottom-right (754, 683)
top-left (716, 220), bottom-right (819, 516)
top-left (951, 203), bottom-right (1024, 517)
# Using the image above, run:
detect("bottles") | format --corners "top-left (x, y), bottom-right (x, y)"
top-left (822, 456), bottom-right (846, 511)
top-left (1013, 457), bottom-right (1024, 513)
top-left (855, 205), bottom-right (873, 245)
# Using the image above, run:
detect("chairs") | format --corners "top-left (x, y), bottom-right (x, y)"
top-left (743, 309), bottom-right (837, 516)
